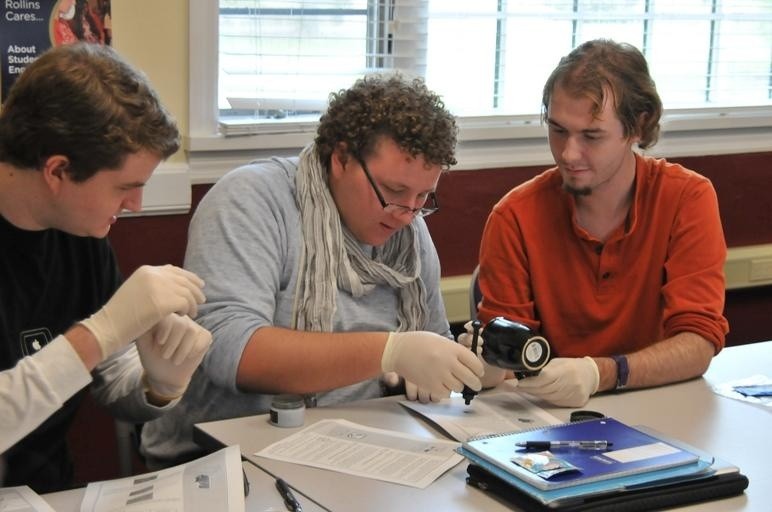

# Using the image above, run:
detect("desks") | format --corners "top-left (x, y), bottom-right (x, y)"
top-left (0, 340), bottom-right (772, 512)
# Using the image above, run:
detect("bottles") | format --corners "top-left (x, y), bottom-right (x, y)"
top-left (270, 393), bottom-right (306, 428)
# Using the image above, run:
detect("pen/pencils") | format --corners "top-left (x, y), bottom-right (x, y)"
top-left (515, 440), bottom-right (613, 451)
top-left (275, 479), bottom-right (302, 512)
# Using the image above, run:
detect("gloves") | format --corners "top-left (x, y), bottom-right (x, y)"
top-left (75, 264), bottom-right (212, 398)
top-left (381, 321), bottom-right (506, 403)
top-left (515, 356), bottom-right (600, 407)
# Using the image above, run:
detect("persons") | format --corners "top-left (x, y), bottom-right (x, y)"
top-left (0, 41), bottom-right (214, 496)
top-left (140, 75), bottom-right (485, 473)
top-left (458, 38), bottom-right (731, 411)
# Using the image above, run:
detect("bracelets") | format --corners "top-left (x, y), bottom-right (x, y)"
top-left (608, 352), bottom-right (629, 393)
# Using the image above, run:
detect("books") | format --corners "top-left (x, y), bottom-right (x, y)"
top-left (453, 417), bottom-right (749, 512)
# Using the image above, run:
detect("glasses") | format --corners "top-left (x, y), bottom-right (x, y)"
top-left (352, 150), bottom-right (439, 218)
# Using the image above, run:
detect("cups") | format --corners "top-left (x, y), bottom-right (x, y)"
top-left (481, 316), bottom-right (551, 372)
